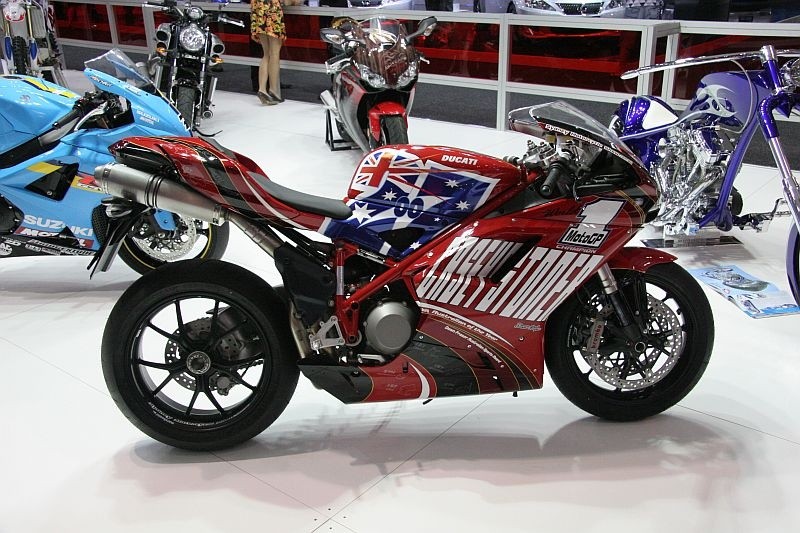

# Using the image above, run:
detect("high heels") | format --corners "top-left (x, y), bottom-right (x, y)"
top-left (258, 92), bottom-right (278, 105)
top-left (269, 91), bottom-right (284, 102)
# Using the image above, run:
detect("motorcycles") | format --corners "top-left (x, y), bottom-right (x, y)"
top-left (0, 0), bottom-right (71, 92)
top-left (143, 0), bottom-right (245, 133)
top-left (320, 16), bottom-right (437, 156)
top-left (86, 98), bottom-right (716, 452)
top-left (0, 46), bottom-right (229, 276)
top-left (501, 45), bottom-right (800, 310)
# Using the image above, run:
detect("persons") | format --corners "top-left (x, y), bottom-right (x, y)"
top-left (250, 0), bottom-right (287, 106)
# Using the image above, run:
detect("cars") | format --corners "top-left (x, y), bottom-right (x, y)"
top-left (308, 0), bottom-right (674, 54)
